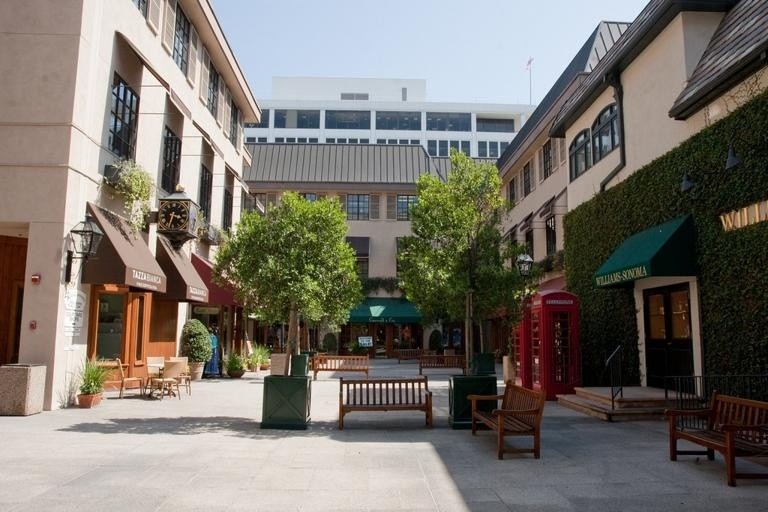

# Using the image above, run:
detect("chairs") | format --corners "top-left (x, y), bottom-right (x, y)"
top-left (116, 357), bottom-right (192, 401)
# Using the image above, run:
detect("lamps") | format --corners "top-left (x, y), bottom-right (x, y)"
top-left (681, 163), bottom-right (721, 194)
top-left (725, 137), bottom-right (756, 169)
top-left (65, 211), bottom-right (104, 283)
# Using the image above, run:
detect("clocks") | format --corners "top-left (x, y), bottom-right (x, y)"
top-left (156, 201), bottom-right (191, 231)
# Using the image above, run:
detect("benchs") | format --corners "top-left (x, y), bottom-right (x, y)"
top-left (312, 354), bottom-right (369, 381)
top-left (664, 389), bottom-right (768, 487)
top-left (418, 354), bottom-right (467, 375)
top-left (398, 349), bottom-right (421, 363)
top-left (467, 379), bottom-right (547, 460)
top-left (339, 376), bottom-right (433, 431)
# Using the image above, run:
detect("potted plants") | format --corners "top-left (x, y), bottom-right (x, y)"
top-left (103, 156), bottom-right (155, 242)
top-left (227, 342), bottom-right (271, 378)
top-left (76, 356), bottom-right (115, 409)
top-left (180, 319), bottom-right (213, 381)
